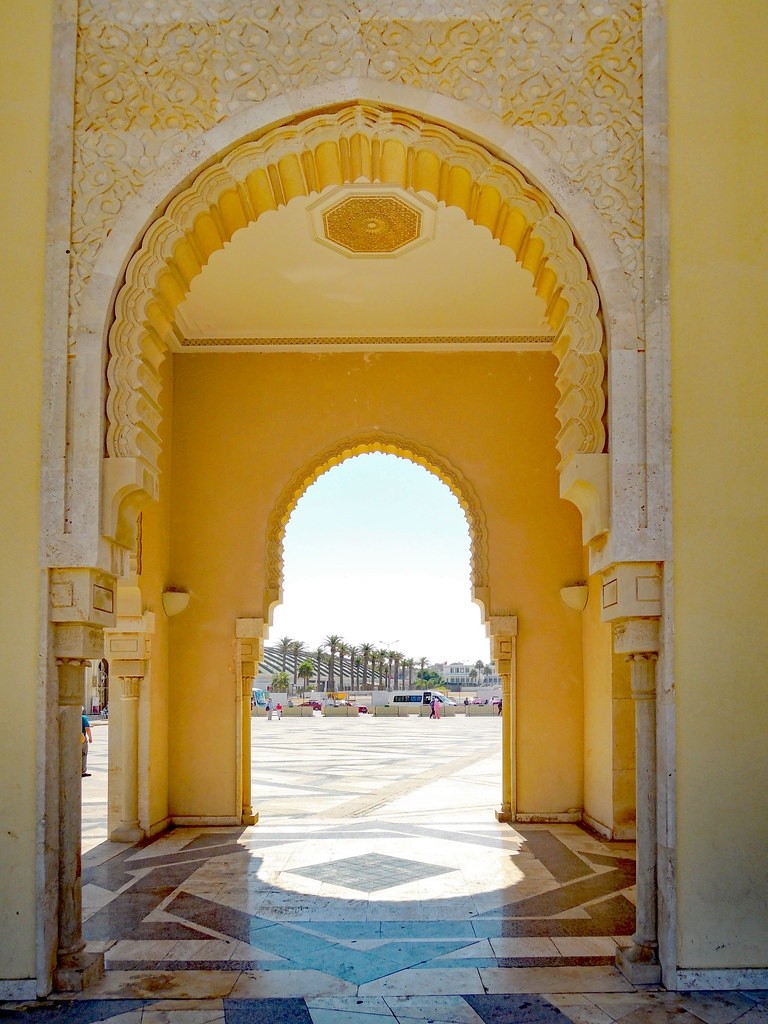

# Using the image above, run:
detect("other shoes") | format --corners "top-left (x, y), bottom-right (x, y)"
top-left (82, 773), bottom-right (91, 777)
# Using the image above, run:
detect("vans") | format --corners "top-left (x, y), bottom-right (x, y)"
top-left (388, 689), bottom-right (458, 708)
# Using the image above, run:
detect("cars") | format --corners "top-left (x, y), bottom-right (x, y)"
top-left (471, 696), bottom-right (501, 706)
top-left (358, 706), bottom-right (368, 713)
top-left (297, 701), bottom-right (321, 711)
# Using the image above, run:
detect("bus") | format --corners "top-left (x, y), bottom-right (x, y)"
top-left (251, 688), bottom-right (270, 706)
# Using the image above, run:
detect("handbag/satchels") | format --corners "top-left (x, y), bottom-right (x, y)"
top-left (266, 706), bottom-right (269, 711)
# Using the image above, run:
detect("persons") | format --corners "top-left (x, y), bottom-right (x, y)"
top-left (265, 698), bottom-right (273, 721)
top-left (429, 697), bottom-right (441, 720)
top-left (496, 698), bottom-right (502, 716)
top-left (484, 699), bottom-right (488, 704)
top-left (464, 698), bottom-right (469, 705)
top-left (275, 703), bottom-right (282, 720)
top-left (82, 706), bottom-right (93, 777)
